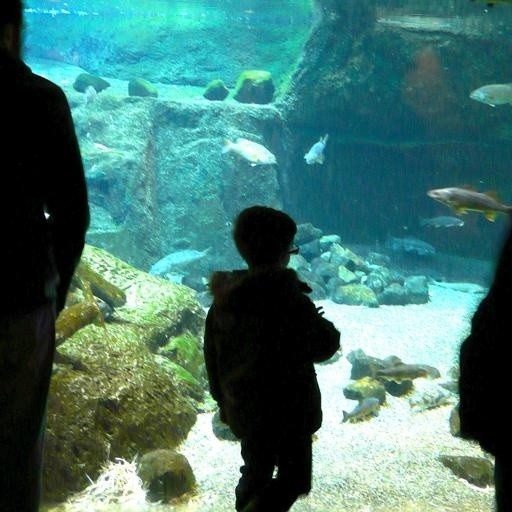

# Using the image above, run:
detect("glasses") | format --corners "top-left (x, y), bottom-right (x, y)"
top-left (285, 244), bottom-right (299, 257)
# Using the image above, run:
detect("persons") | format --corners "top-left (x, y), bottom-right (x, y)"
top-left (456, 205), bottom-right (511, 512)
top-left (202, 203), bottom-right (344, 512)
top-left (0, 0), bottom-right (92, 512)
